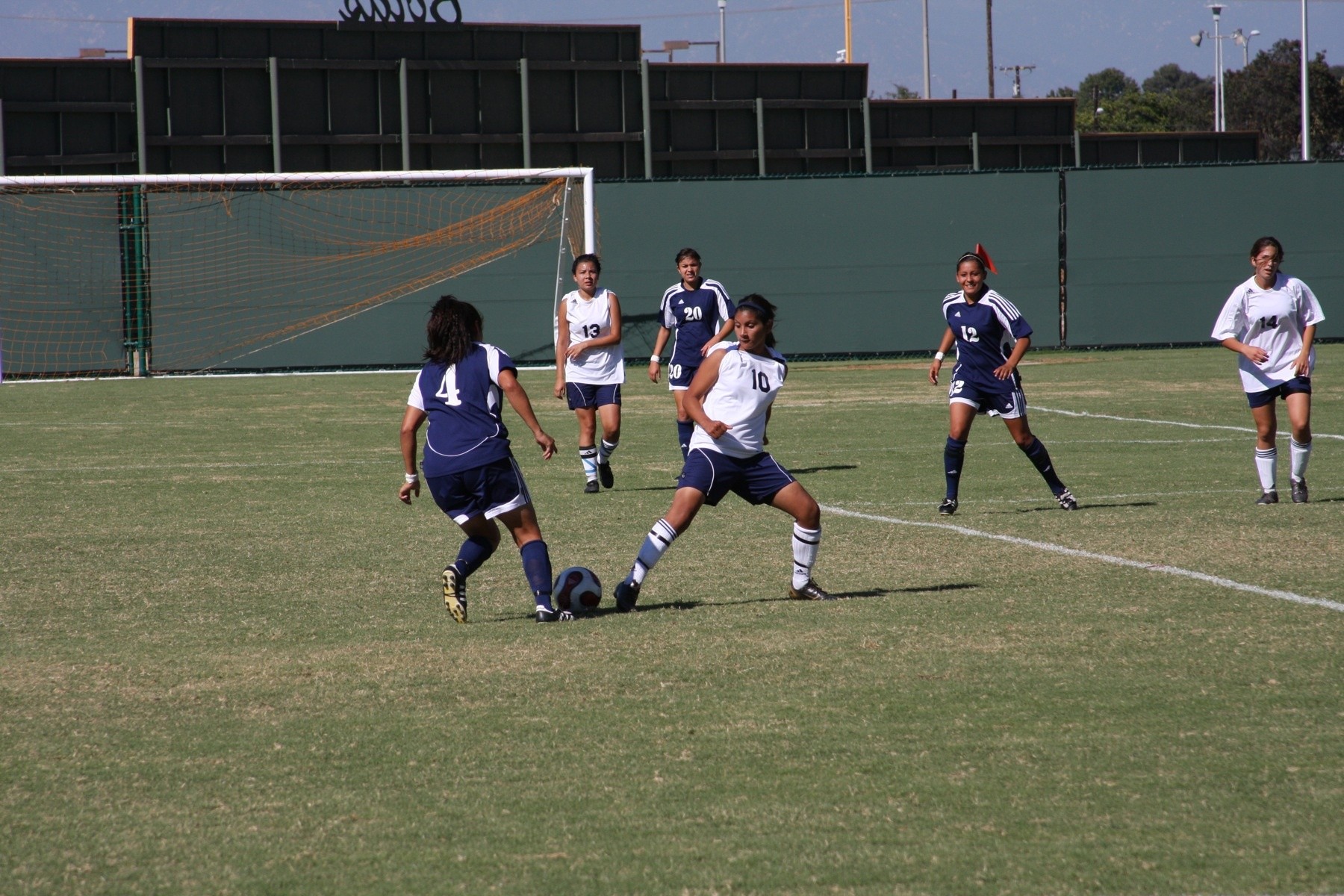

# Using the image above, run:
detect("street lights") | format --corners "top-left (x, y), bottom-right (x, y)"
top-left (1189, 3), bottom-right (1260, 132)
top-left (663, 39), bottom-right (721, 62)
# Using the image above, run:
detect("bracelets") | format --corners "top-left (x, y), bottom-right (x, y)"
top-left (405, 470), bottom-right (419, 484)
top-left (650, 354), bottom-right (660, 364)
top-left (934, 351), bottom-right (946, 363)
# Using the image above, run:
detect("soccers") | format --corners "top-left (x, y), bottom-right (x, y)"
top-left (554, 567), bottom-right (602, 614)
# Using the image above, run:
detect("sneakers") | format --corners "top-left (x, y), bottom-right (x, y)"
top-left (536, 608), bottom-right (577, 624)
top-left (613, 579), bottom-right (642, 613)
top-left (442, 564), bottom-right (467, 624)
top-left (939, 498), bottom-right (958, 515)
top-left (788, 577), bottom-right (838, 602)
top-left (1256, 491), bottom-right (1278, 505)
top-left (1053, 485), bottom-right (1077, 511)
top-left (584, 480), bottom-right (599, 493)
top-left (597, 460), bottom-right (614, 488)
top-left (1289, 472), bottom-right (1308, 503)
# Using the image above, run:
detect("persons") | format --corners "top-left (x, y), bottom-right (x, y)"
top-left (399, 295), bottom-right (575, 625)
top-left (928, 252), bottom-right (1077, 517)
top-left (554, 254), bottom-right (625, 492)
top-left (648, 248), bottom-right (737, 464)
top-left (613, 292), bottom-right (838, 613)
top-left (1211, 235), bottom-right (1324, 504)
